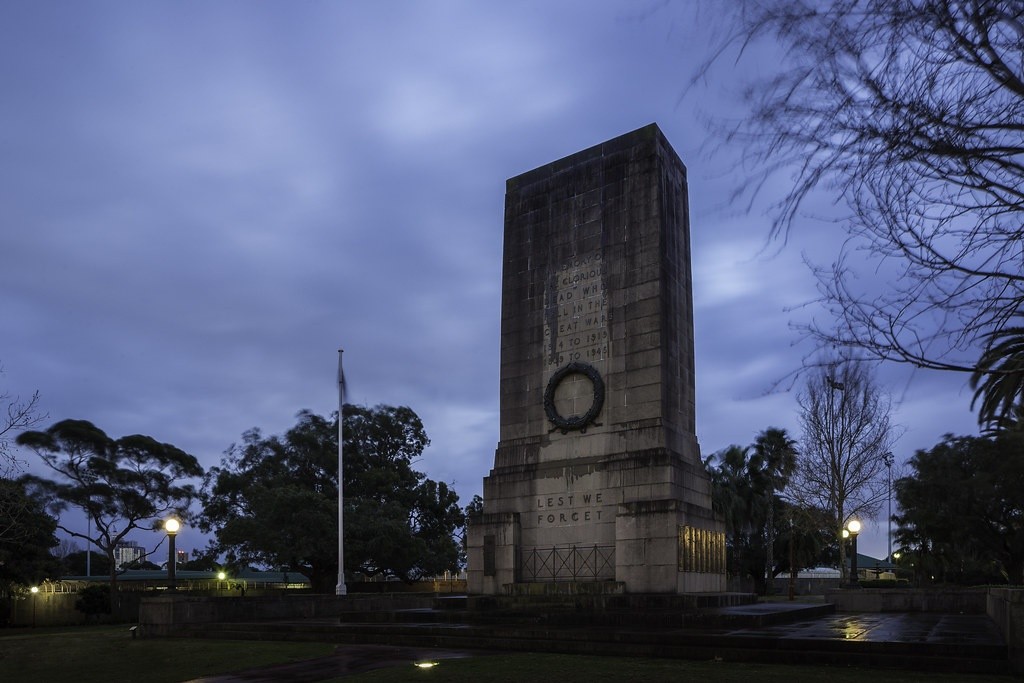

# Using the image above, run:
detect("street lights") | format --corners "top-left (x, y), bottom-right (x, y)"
top-left (162, 516), bottom-right (183, 590)
top-left (882, 451), bottom-right (895, 568)
top-left (848, 519), bottom-right (861, 584)
top-left (840, 529), bottom-right (850, 583)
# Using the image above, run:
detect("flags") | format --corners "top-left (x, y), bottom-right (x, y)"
top-left (342, 370), bottom-right (346, 397)
